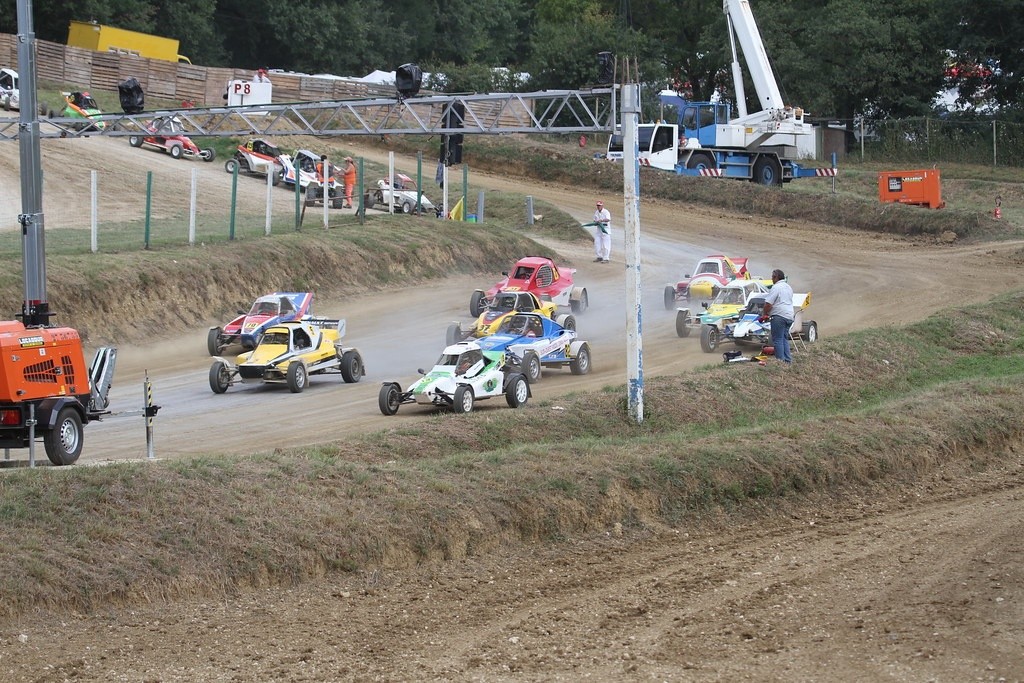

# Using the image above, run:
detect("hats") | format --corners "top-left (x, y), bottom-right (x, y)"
top-left (343, 156), bottom-right (354, 162)
top-left (596, 201), bottom-right (603, 206)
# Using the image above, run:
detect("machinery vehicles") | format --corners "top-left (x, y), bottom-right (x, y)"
top-left (0, 0), bottom-right (116, 464)
top-left (676, 0), bottom-right (811, 148)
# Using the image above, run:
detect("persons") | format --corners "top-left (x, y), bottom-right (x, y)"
top-left (592, 200), bottom-right (611, 264)
top-left (506, 317), bottom-right (539, 338)
top-left (161, 120), bottom-right (172, 132)
top-left (253, 69), bottom-right (271, 83)
top-left (1, 76), bottom-right (16, 90)
top-left (303, 158), bottom-right (316, 173)
top-left (496, 297), bottom-right (524, 312)
top-left (763, 269), bottom-right (794, 367)
top-left (450, 354), bottom-right (472, 377)
top-left (514, 265), bottom-right (545, 288)
top-left (258, 301), bottom-right (305, 349)
top-left (341, 157), bottom-right (356, 208)
top-left (259, 144), bottom-right (267, 155)
top-left (315, 155), bottom-right (338, 186)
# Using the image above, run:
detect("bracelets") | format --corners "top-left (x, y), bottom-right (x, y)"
top-left (601, 219), bottom-right (603, 222)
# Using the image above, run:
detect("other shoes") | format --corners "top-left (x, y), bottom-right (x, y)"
top-left (592, 258), bottom-right (603, 262)
top-left (343, 203), bottom-right (352, 208)
top-left (601, 260), bottom-right (610, 264)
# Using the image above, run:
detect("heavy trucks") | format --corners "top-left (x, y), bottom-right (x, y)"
top-left (594, 123), bottom-right (838, 194)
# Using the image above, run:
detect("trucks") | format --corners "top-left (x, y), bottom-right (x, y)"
top-left (66, 20), bottom-right (193, 65)
